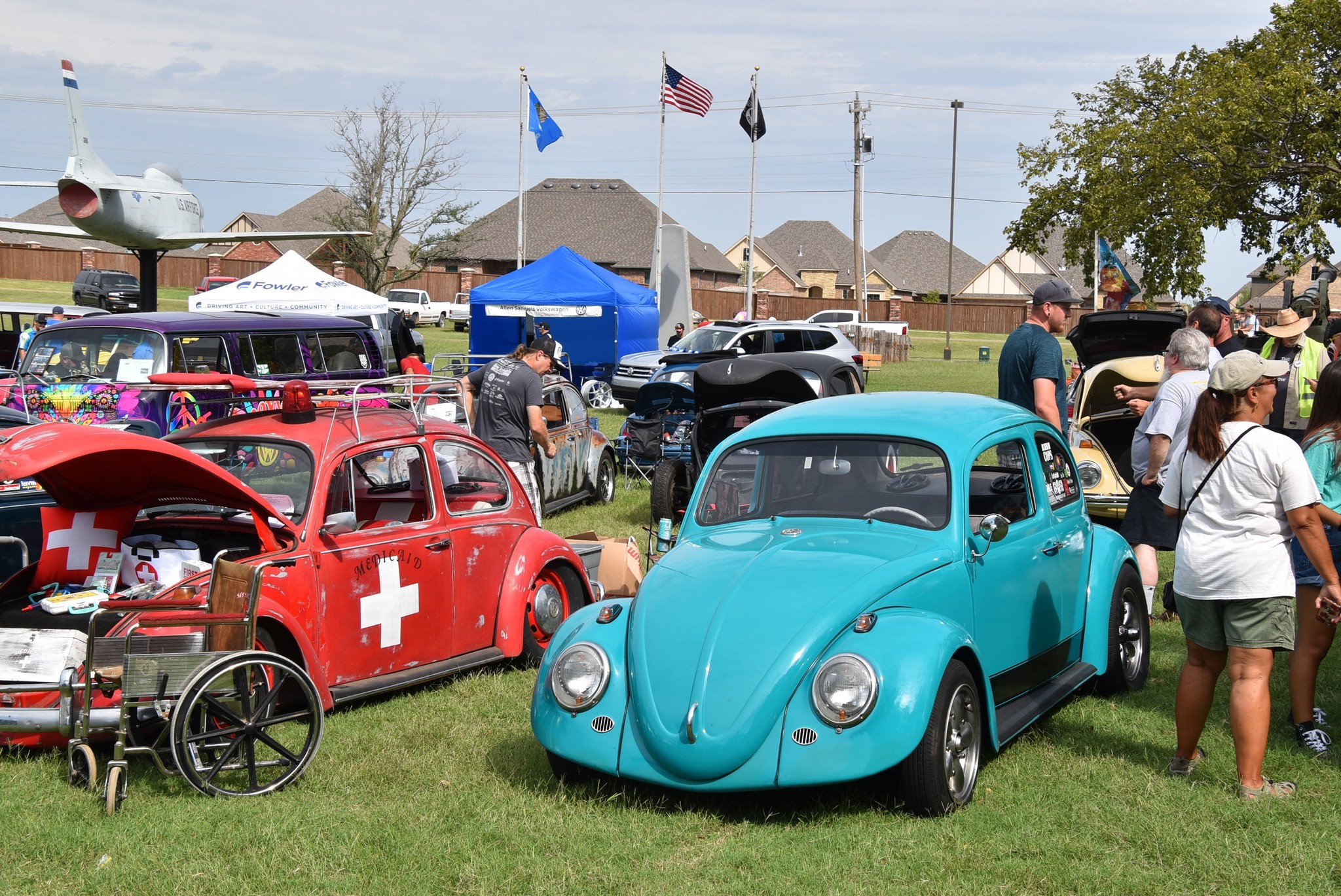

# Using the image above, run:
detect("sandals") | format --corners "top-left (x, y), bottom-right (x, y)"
top-left (1168, 745), bottom-right (1206, 776)
top-left (1239, 774), bottom-right (1298, 805)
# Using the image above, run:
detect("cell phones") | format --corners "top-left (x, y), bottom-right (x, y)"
top-left (1316, 597), bottom-right (1341, 629)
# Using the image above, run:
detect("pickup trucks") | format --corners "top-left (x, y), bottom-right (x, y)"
top-left (193, 277), bottom-right (239, 296)
top-left (788, 310), bottom-right (910, 351)
top-left (447, 292), bottom-right (470, 332)
top-left (386, 289), bottom-right (451, 328)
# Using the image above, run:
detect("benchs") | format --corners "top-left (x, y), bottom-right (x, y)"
top-left (858, 348), bottom-right (883, 385)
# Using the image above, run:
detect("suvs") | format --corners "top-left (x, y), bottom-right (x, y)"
top-left (612, 319), bottom-right (867, 414)
top-left (72, 267), bottom-right (139, 314)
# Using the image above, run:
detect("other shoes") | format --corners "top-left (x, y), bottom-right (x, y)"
top-left (1156, 611), bottom-right (1179, 622)
top-left (1149, 614), bottom-right (1154, 626)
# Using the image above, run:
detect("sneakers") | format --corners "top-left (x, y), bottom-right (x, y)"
top-left (1295, 721), bottom-right (1336, 763)
top-left (1287, 703), bottom-right (1331, 731)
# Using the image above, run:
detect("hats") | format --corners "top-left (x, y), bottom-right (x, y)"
top-left (1202, 296), bottom-right (1230, 314)
top-left (52, 306), bottom-right (65, 314)
top-left (1327, 319), bottom-right (1341, 337)
top-left (1208, 348), bottom-right (1290, 391)
top-left (535, 322), bottom-right (550, 330)
top-left (34, 314), bottom-right (48, 324)
top-left (1258, 307), bottom-right (1315, 338)
top-left (60, 341), bottom-right (86, 362)
top-left (674, 323), bottom-right (685, 332)
top-left (1032, 280), bottom-right (1084, 306)
top-left (530, 338), bottom-right (567, 371)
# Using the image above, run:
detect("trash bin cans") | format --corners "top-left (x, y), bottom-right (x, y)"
top-left (944, 347), bottom-right (951, 359)
top-left (979, 346), bottom-right (990, 362)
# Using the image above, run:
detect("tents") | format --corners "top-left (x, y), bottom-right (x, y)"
top-left (468, 245), bottom-right (659, 389)
top-left (189, 249), bottom-right (390, 381)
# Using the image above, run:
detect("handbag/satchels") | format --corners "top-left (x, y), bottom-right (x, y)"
top-left (1162, 581), bottom-right (1177, 611)
top-left (120, 530), bottom-right (202, 597)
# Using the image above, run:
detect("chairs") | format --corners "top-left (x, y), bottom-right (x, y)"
top-left (776, 508), bottom-right (865, 516)
top-left (908, 514), bottom-right (1012, 535)
top-left (624, 416), bottom-right (667, 490)
top-left (357, 518), bottom-right (404, 531)
top-left (588, 415), bottom-right (626, 450)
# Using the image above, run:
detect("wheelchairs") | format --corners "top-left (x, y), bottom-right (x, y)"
top-left (68, 545), bottom-right (323, 818)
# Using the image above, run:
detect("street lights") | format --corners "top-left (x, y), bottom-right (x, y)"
top-left (946, 99), bottom-right (965, 349)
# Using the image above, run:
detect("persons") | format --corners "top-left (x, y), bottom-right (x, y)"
top-left (994, 271), bottom-right (1076, 488)
top-left (667, 323), bottom-right (691, 353)
top-left (1111, 296), bottom-right (1341, 804)
top-left (534, 323), bottom-right (551, 339)
top-left (49, 342), bottom-right (86, 377)
top-left (17, 306), bottom-right (69, 370)
top-left (456, 332), bottom-right (562, 538)
top-left (401, 353), bottom-right (438, 407)
top-left (697, 318), bottom-right (713, 350)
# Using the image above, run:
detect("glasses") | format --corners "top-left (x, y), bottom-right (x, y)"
top-left (1242, 376), bottom-right (1279, 397)
top-left (1197, 301), bottom-right (1217, 309)
top-left (1181, 320), bottom-right (1198, 329)
top-left (538, 327), bottom-right (542, 330)
top-left (38, 322), bottom-right (47, 325)
top-left (1161, 349), bottom-right (1168, 356)
top-left (1330, 335), bottom-right (1340, 342)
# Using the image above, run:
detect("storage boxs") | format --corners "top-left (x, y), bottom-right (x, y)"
top-left (567, 543), bottom-right (604, 599)
top-left (563, 531), bottom-right (642, 600)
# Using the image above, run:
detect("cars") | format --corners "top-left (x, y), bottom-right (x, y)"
top-left (413, 355), bottom-right (615, 518)
top-left (1065, 311), bottom-right (1190, 519)
top-left (0, 379), bottom-right (603, 745)
top-left (529, 390), bottom-right (1150, 817)
top-left (610, 351), bottom-right (900, 528)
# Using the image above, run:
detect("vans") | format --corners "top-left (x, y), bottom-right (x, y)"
top-left (0, 301), bottom-right (112, 371)
top-left (6, 309), bottom-right (393, 480)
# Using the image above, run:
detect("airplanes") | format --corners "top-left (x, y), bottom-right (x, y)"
top-left (0, 150), bottom-right (374, 250)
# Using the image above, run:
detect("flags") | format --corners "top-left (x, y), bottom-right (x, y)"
top-left (1099, 234), bottom-right (1141, 310)
top-left (739, 76), bottom-right (767, 142)
top-left (527, 85), bottom-right (564, 153)
top-left (660, 62), bottom-right (713, 118)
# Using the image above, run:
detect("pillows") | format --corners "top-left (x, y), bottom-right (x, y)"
top-left (29, 502), bottom-right (142, 594)
